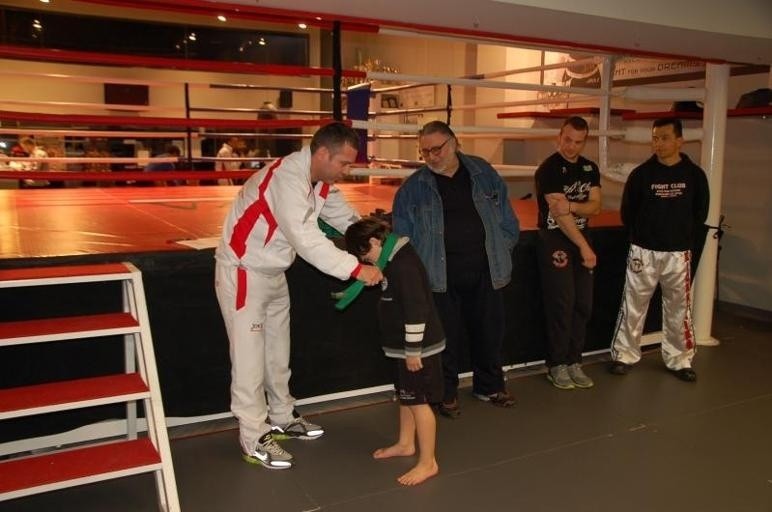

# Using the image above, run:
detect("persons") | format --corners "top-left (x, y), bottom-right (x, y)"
top-left (214, 136), bottom-right (240, 185)
top-left (392, 120), bottom-right (521, 418)
top-left (9, 134), bottom-right (50, 188)
top-left (214, 121), bottom-right (384, 471)
top-left (608, 117), bottom-right (710, 383)
top-left (533, 117), bottom-right (601, 389)
top-left (145, 146), bottom-right (180, 185)
top-left (344, 215), bottom-right (447, 486)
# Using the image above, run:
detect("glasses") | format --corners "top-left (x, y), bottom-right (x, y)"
top-left (418, 139), bottom-right (450, 156)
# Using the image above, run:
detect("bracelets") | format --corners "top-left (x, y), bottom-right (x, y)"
top-left (568, 199), bottom-right (572, 214)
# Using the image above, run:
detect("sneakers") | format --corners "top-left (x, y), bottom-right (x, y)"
top-left (472, 390), bottom-right (517, 408)
top-left (670, 367), bottom-right (695, 382)
top-left (240, 432), bottom-right (295, 470)
top-left (608, 361), bottom-right (633, 374)
top-left (547, 364), bottom-right (575, 389)
top-left (568, 363), bottom-right (594, 388)
top-left (266, 410), bottom-right (325, 440)
top-left (434, 397), bottom-right (464, 419)
